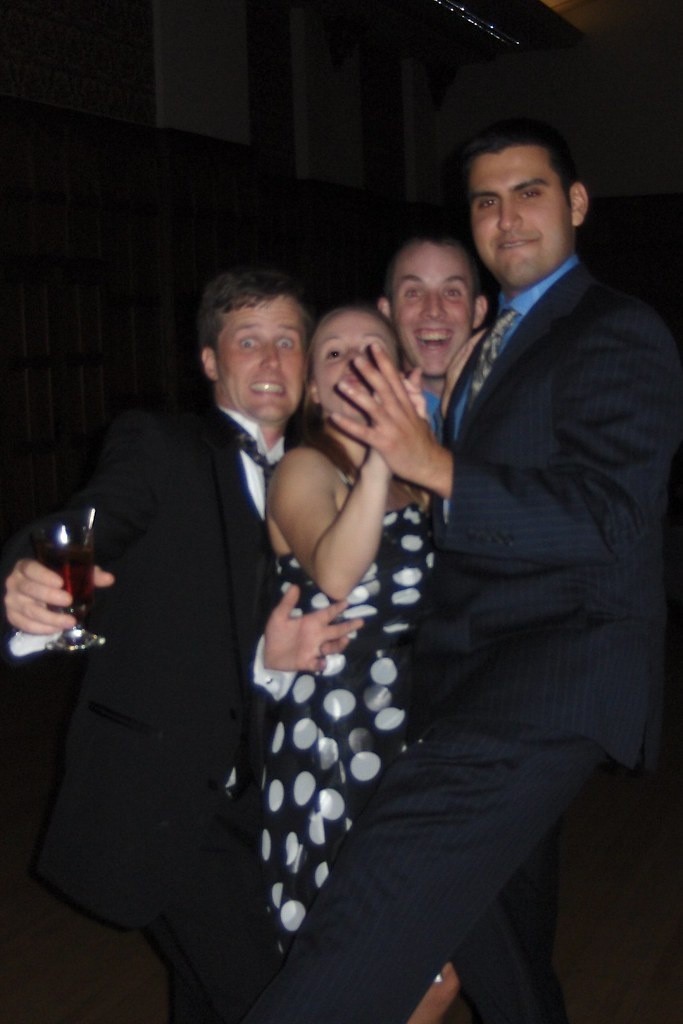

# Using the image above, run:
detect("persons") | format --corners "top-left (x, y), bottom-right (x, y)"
top-left (0, 115), bottom-right (683, 1023)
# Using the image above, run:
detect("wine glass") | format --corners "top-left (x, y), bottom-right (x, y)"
top-left (27, 522), bottom-right (108, 651)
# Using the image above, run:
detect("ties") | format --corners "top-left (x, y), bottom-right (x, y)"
top-left (226, 412), bottom-right (284, 520)
top-left (462, 306), bottom-right (521, 417)
